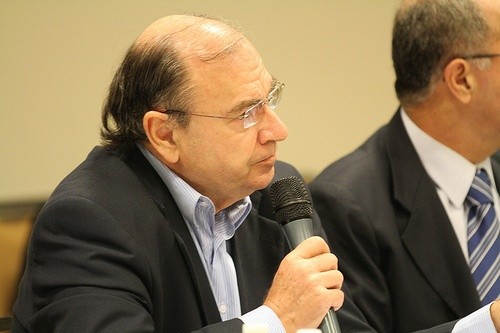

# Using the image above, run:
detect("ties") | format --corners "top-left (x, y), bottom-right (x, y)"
top-left (464, 167), bottom-right (500, 304)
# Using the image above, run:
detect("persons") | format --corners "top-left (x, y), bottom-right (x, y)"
top-left (9, 14), bottom-right (500, 333)
top-left (309, 0), bottom-right (500, 333)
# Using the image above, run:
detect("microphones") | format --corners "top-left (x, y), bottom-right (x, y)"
top-left (268, 176), bottom-right (342, 333)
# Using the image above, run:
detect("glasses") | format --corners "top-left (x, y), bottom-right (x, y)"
top-left (163, 79), bottom-right (285, 130)
